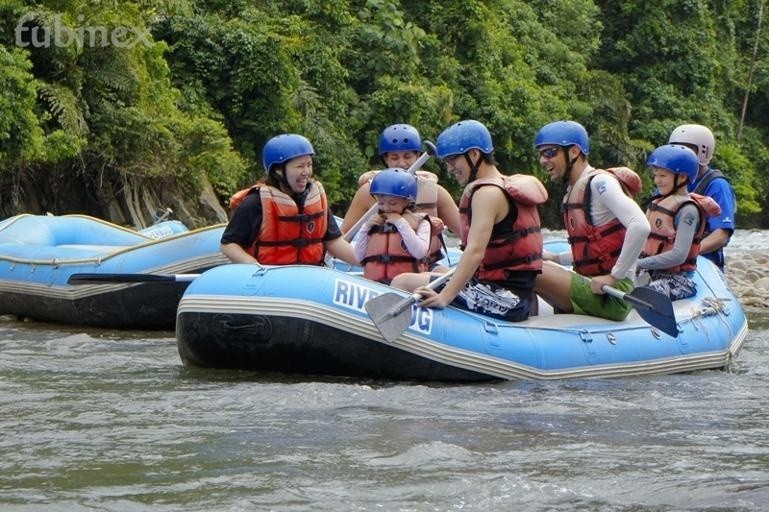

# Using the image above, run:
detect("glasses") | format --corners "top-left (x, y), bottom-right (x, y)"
top-left (538, 147), bottom-right (559, 159)
top-left (444, 154), bottom-right (462, 165)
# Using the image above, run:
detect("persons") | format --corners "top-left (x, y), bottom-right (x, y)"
top-left (390, 120), bottom-right (549, 324)
top-left (655, 124), bottom-right (737, 266)
top-left (339, 124), bottom-right (465, 247)
top-left (220, 134), bottom-right (360, 270)
top-left (353, 167), bottom-right (445, 284)
top-left (636, 144), bottom-right (721, 301)
top-left (529, 120), bottom-right (651, 321)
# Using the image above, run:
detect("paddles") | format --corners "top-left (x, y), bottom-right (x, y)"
top-left (67, 273), bottom-right (199, 286)
top-left (542, 260), bottom-right (678, 338)
top-left (365, 265), bottom-right (460, 344)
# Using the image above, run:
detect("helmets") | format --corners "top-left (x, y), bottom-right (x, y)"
top-left (262, 133), bottom-right (315, 173)
top-left (370, 167), bottom-right (418, 203)
top-left (646, 143), bottom-right (700, 184)
top-left (378, 123), bottom-right (423, 156)
top-left (436, 118), bottom-right (495, 159)
top-left (668, 124), bottom-right (715, 167)
top-left (534, 120), bottom-right (590, 155)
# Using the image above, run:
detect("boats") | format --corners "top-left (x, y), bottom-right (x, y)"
top-left (1, 212), bottom-right (347, 329)
top-left (172, 241), bottom-right (750, 384)
